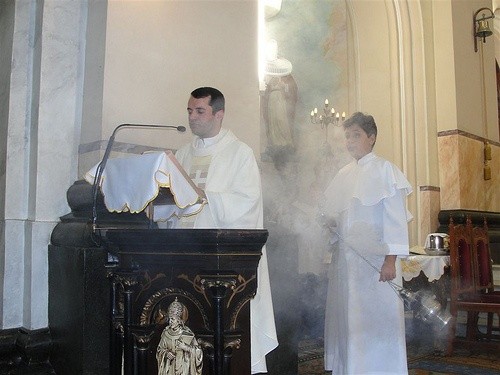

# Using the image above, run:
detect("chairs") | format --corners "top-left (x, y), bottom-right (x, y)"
top-left (446, 216), bottom-right (500, 356)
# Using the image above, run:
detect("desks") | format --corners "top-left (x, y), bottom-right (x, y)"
top-left (402, 255), bottom-right (494, 357)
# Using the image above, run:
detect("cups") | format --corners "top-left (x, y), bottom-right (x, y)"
top-left (430, 236), bottom-right (443, 248)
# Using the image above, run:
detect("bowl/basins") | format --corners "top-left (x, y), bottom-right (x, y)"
top-left (424, 248), bottom-right (450, 256)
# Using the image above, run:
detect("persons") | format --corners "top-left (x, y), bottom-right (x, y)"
top-left (285, 182), bottom-right (337, 342)
top-left (155, 297), bottom-right (203, 375)
top-left (174, 87), bottom-right (278, 374)
top-left (316, 110), bottom-right (414, 375)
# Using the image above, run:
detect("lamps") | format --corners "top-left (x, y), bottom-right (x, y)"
top-left (310, 98), bottom-right (346, 142)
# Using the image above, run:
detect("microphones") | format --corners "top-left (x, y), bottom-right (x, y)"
top-left (92, 124), bottom-right (186, 218)
